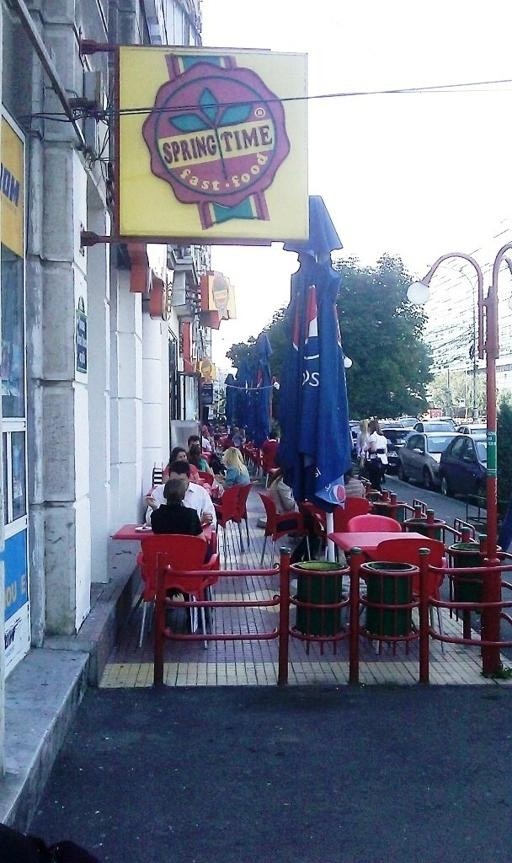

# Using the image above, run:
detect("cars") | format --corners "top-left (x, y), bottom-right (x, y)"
top-left (349, 417), bottom-right (487, 508)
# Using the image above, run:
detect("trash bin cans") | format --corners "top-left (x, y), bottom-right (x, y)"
top-left (374, 501), bottom-right (404, 522)
top-left (364, 561), bottom-right (417, 636)
top-left (451, 542), bottom-right (502, 614)
top-left (295, 561), bottom-right (346, 635)
top-left (406, 519), bottom-right (441, 541)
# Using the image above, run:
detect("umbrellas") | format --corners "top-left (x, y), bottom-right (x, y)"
top-left (223, 372), bottom-right (236, 430)
top-left (234, 358), bottom-right (250, 442)
top-left (247, 331), bottom-right (275, 476)
top-left (273, 193), bottom-right (355, 562)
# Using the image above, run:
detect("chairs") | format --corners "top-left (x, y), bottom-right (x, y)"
top-left (112, 418), bottom-right (449, 655)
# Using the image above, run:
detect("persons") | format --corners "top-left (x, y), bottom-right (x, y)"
top-left (161, 415), bottom-right (279, 519)
top-left (355, 419), bottom-right (370, 478)
top-left (149, 478), bottom-right (214, 630)
top-left (145, 459), bottom-right (219, 532)
top-left (364, 420), bottom-right (389, 493)
top-left (266, 473), bottom-right (322, 564)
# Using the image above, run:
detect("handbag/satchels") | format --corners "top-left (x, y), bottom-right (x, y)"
top-left (364, 458), bottom-right (383, 474)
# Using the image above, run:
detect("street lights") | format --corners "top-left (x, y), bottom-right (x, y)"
top-left (407, 243), bottom-right (512, 672)
top-left (269, 376), bottom-right (280, 432)
